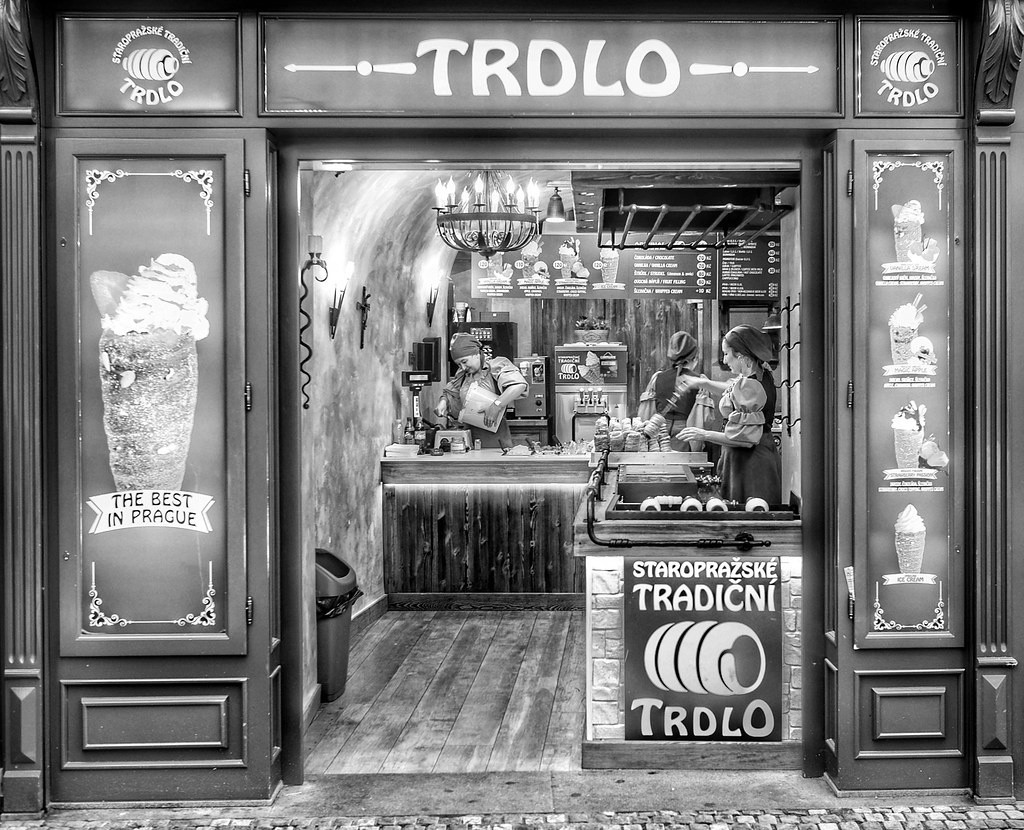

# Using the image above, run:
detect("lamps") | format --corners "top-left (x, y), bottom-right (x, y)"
top-left (545, 184), bottom-right (565, 223)
top-left (433, 168), bottom-right (542, 260)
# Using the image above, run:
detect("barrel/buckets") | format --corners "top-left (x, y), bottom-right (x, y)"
top-left (458, 382), bottom-right (506, 433)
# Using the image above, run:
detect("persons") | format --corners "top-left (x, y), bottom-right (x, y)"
top-left (432, 333), bottom-right (530, 447)
top-left (676, 323), bottom-right (782, 505)
top-left (636, 331), bottom-right (715, 452)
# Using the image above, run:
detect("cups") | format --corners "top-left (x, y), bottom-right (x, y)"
top-left (450, 436), bottom-right (466, 454)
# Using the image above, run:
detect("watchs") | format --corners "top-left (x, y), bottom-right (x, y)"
top-left (494, 399), bottom-right (504, 409)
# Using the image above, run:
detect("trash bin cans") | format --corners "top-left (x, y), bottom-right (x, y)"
top-left (315, 548), bottom-right (357, 703)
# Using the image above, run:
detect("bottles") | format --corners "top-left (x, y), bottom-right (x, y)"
top-left (394, 419), bottom-right (404, 445)
top-left (404, 417), bottom-right (415, 444)
top-left (415, 417), bottom-right (425, 446)
